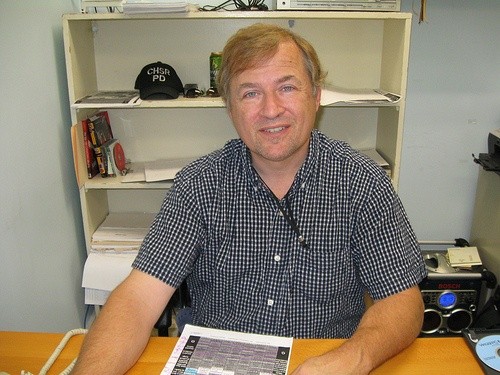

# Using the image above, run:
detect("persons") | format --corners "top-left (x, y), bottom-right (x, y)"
top-left (70, 22), bottom-right (428, 375)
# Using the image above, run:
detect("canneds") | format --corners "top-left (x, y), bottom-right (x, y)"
top-left (209, 52), bottom-right (222, 88)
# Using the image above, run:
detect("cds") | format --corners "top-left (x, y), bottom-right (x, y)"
top-left (475, 334), bottom-right (500, 372)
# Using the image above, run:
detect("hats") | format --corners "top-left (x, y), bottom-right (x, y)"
top-left (135, 61), bottom-right (183, 99)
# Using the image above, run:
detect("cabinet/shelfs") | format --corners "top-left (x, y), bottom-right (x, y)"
top-left (469, 160), bottom-right (500, 286)
top-left (59, 9), bottom-right (416, 318)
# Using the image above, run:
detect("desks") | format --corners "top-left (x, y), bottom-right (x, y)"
top-left (0, 325), bottom-right (486, 375)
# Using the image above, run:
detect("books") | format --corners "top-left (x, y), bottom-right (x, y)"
top-left (82, 111), bottom-right (129, 178)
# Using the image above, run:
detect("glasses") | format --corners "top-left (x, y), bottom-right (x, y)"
top-left (184, 87), bottom-right (220, 98)
